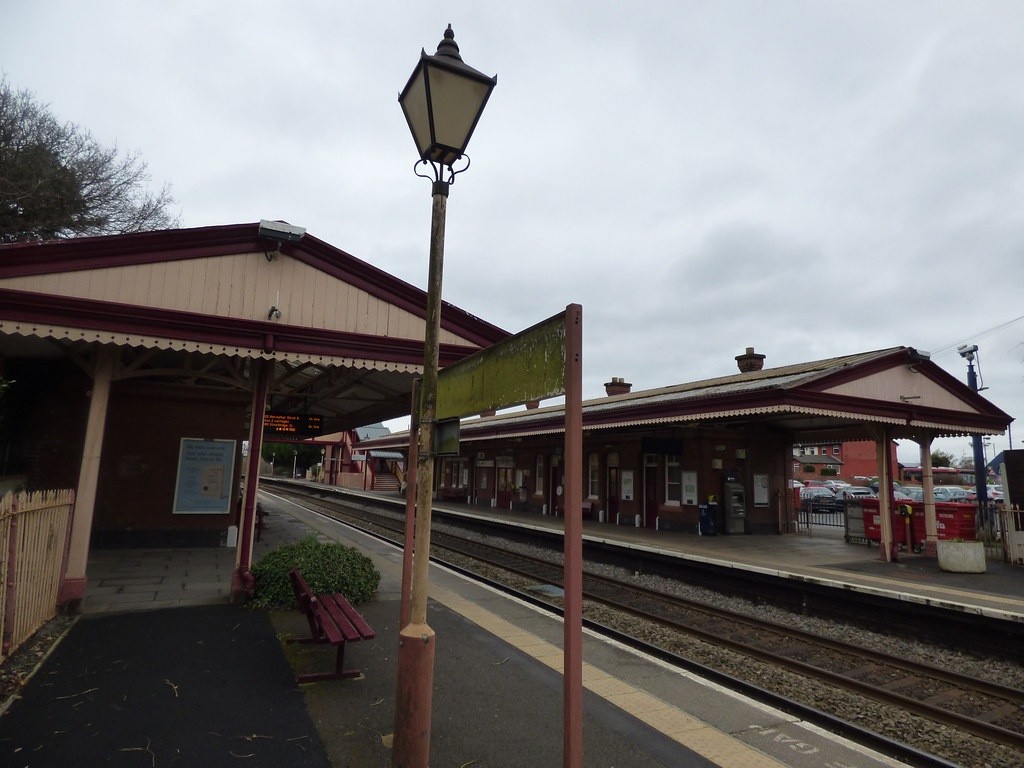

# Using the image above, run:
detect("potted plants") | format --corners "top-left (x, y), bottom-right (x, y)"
top-left (936, 539), bottom-right (986, 572)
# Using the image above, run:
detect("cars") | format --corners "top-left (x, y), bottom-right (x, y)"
top-left (792, 480), bottom-right (1004, 511)
top-left (800, 487), bottom-right (837, 514)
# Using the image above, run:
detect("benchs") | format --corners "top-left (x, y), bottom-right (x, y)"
top-left (284, 563), bottom-right (376, 682)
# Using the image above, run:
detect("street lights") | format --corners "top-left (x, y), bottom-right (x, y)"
top-left (271, 453), bottom-right (275, 477)
top-left (318, 446), bottom-right (326, 483)
top-left (293, 449), bottom-right (298, 478)
top-left (395, 22), bottom-right (500, 767)
